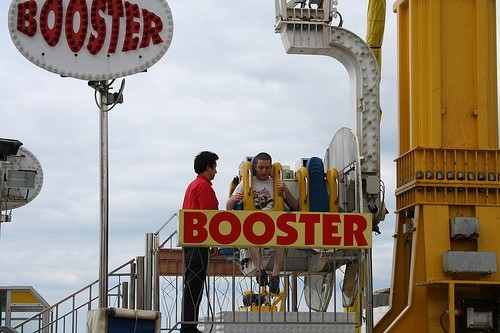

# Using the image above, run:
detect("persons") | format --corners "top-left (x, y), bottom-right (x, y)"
top-left (180, 151), bottom-right (219, 333)
top-left (226, 153), bottom-right (298, 296)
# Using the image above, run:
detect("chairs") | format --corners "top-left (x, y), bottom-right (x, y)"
top-left (229, 158), bottom-right (343, 250)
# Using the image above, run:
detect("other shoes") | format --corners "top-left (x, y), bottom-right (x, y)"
top-left (269, 275), bottom-right (279, 294)
top-left (257, 269), bottom-right (268, 286)
top-left (180, 328), bottom-right (202, 333)
